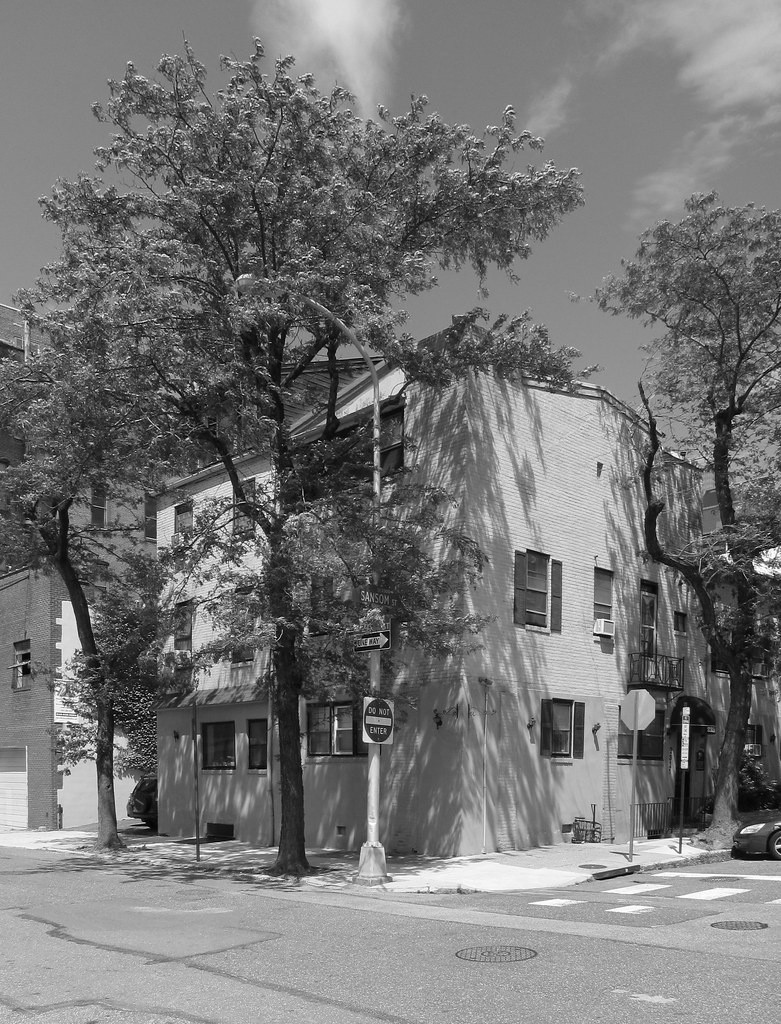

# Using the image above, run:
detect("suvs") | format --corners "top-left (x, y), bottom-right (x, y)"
top-left (126, 774), bottom-right (158, 831)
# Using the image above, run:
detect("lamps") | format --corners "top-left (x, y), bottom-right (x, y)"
top-left (592, 723), bottom-right (601, 735)
top-left (770, 733), bottom-right (776, 745)
top-left (527, 718), bottom-right (536, 729)
top-left (432, 704), bottom-right (458, 730)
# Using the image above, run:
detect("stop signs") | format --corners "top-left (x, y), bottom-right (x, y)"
top-left (362, 696), bottom-right (395, 745)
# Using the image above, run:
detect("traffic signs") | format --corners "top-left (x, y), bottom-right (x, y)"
top-left (346, 629), bottom-right (391, 651)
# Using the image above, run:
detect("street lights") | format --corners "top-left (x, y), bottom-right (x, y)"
top-left (235, 272), bottom-right (395, 889)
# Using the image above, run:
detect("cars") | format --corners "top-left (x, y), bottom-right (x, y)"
top-left (733, 816), bottom-right (781, 864)
